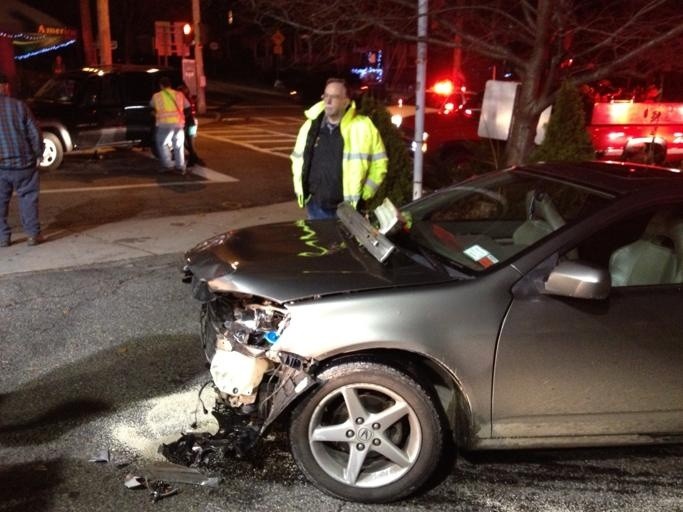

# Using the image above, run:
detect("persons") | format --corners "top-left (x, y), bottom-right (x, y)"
top-left (579, 73), bottom-right (666, 104)
top-left (59, 78), bottom-right (77, 102)
top-left (289, 78), bottom-right (390, 221)
top-left (170, 76), bottom-right (201, 167)
top-left (293, 209), bottom-right (414, 265)
top-left (0, 75), bottom-right (45, 246)
top-left (149, 76), bottom-right (190, 176)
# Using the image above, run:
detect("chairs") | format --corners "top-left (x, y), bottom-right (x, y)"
top-left (527, 190), bottom-right (683, 286)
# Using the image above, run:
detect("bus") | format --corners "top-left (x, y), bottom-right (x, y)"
top-left (587, 99), bottom-right (683, 161)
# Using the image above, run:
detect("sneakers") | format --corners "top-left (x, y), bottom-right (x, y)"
top-left (28, 234), bottom-right (45, 247)
top-left (0, 240), bottom-right (10, 248)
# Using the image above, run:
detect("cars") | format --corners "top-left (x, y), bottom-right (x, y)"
top-left (25, 65), bottom-right (198, 171)
top-left (185, 160), bottom-right (683, 505)
